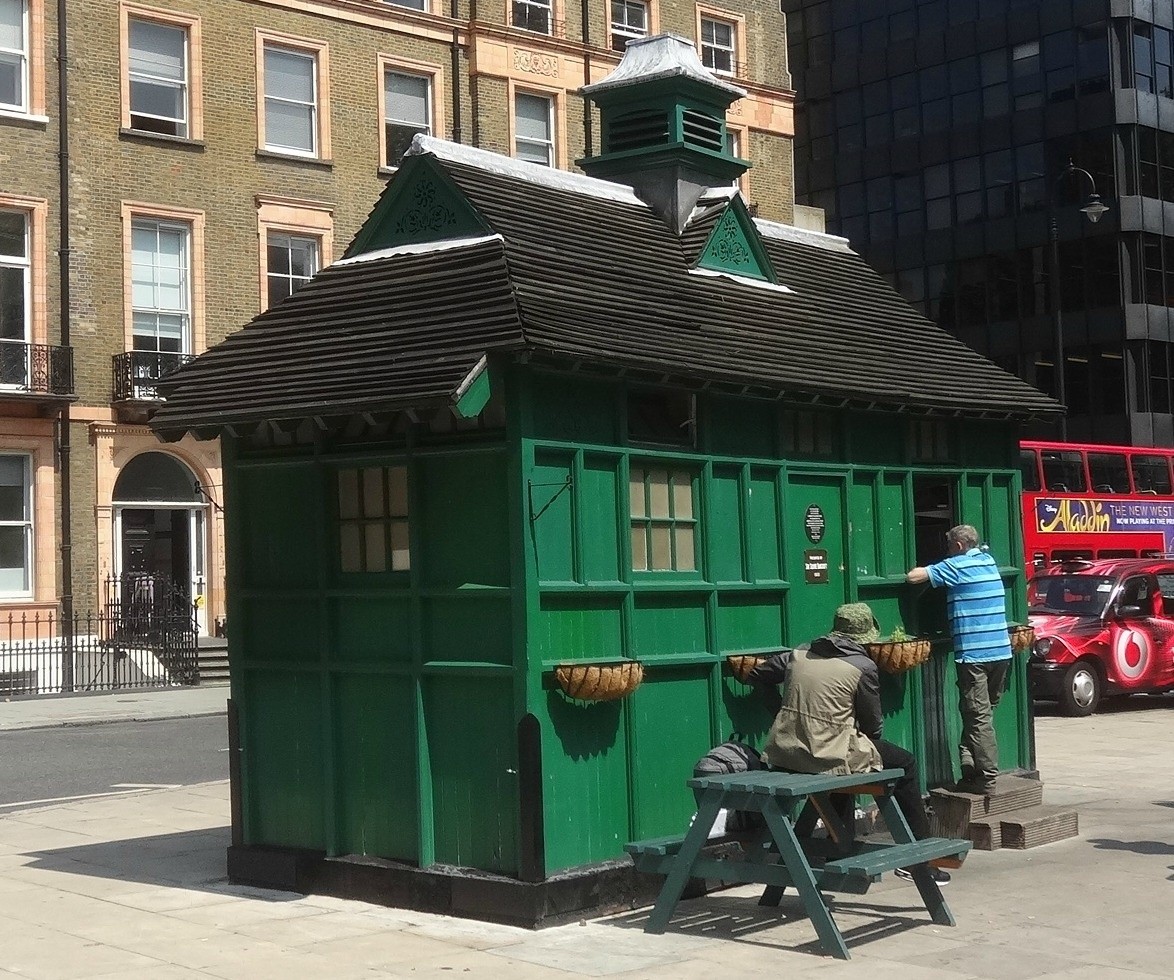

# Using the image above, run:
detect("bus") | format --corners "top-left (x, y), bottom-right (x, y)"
top-left (1018, 439), bottom-right (1174, 582)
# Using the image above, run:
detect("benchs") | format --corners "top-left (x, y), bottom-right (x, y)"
top-left (824, 838), bottom-right (974, 877)
top-left (623, 828), bottom-right (773, 856)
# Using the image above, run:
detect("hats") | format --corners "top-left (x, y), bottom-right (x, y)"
top-left (828, 602), bottom-right (881, 646)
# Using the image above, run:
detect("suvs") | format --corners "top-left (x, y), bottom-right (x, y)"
top-left (1023, 560), bottom-right (1174, 718)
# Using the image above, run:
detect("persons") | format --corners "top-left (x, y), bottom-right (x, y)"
top-left (905, 525), bottom-right (1012, 796)
top-left (746, 600), bottom-right (952, 887)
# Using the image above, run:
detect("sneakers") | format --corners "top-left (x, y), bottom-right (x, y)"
top-left (894, 867), bottom-right (951, 887)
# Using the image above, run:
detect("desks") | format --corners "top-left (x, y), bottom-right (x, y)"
top-left (630, 768), bottom-right (968, 960)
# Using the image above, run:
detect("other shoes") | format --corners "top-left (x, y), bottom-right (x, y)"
top-left (956, 773), bottom-right (996, 796)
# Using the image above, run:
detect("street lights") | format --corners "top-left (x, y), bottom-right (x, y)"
top-left (1044, 167), bottom-right (1109, 440)
